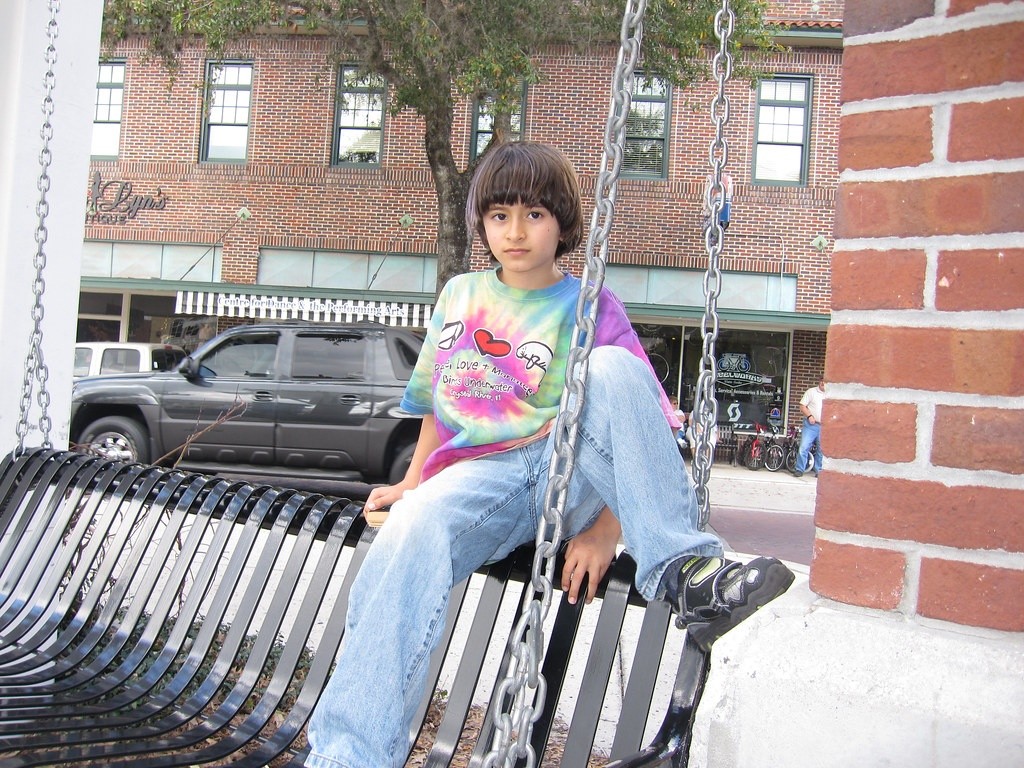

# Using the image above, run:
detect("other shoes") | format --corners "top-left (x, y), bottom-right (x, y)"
top-left (672, 552), bottom-right (798, 652)
top-left (793, 470), bottom-right (802, 477)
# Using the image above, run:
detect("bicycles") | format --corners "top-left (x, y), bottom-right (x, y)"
top-left (741, 418), bottom-right (818, 473)
top-left (749, 345), bottom-right (787, 418)
top-left (633, 323), bottom-right (671, 386)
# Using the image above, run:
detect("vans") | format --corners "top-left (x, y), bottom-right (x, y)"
top-left (73, 340), bottom-right (187, 381)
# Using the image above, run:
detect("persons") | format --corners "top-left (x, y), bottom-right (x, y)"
top-left (301, 143), bottom-right (824, 768)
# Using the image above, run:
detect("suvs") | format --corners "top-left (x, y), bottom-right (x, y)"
top-left (68, 317), bottom-right (428, 486)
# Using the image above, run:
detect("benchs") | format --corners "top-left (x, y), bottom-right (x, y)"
top-left (0, 447), bottom-right (713, 768)
